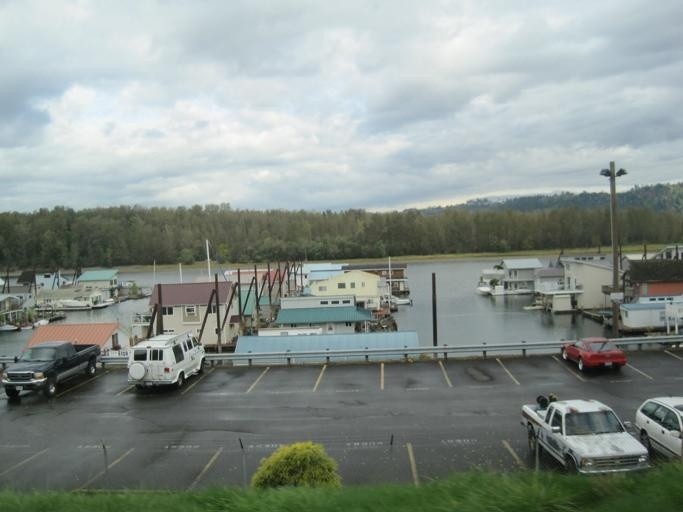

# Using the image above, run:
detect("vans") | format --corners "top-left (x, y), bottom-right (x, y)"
top-left (127, 329), bottom-right (206, 391)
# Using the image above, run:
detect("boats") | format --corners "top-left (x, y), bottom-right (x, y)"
top-left (387, 295), bottom-right (410, 305)
top-left (523, 302), bottom-right (544, 310)
top-left (0, 324), bottom-right (18, 333)
top-left (474, 282), bottom-right (490, 296)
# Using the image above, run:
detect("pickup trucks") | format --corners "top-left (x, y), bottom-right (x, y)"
top-left (0, 341), bottom-right (100, 397)
top-left (521, 398), bottom-right (652, 477)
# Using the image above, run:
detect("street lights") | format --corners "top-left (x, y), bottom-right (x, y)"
top-left (598, 160), bottom-right (627, 335)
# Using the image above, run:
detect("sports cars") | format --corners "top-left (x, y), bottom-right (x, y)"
top-left (560, 336), bottom-right (627, 374)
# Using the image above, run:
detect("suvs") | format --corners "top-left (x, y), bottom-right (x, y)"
top-left (634, 396), bottom-right (683, 463)
